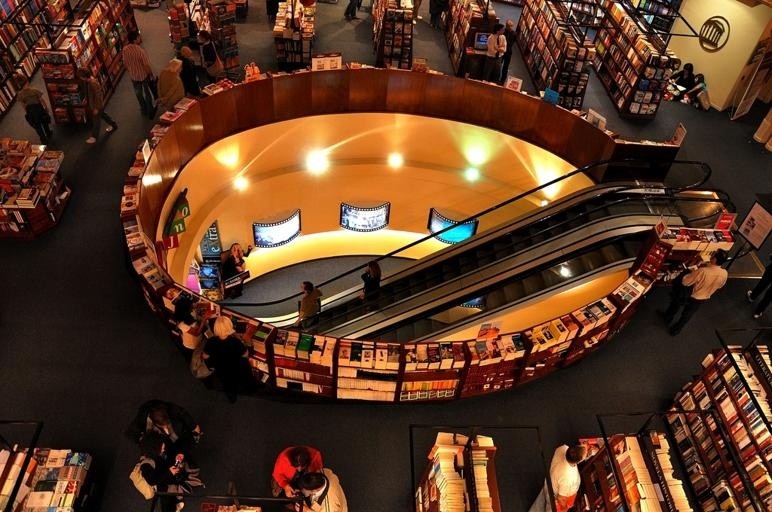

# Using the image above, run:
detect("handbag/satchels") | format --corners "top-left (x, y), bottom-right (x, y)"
top-left (190, 350), bottom-right (215, 379)
top-left (696, 90), bottom-right (710, 111)
top-left (207, 57), bottom-right (224, 76)
top-left (301, 315), bottom-right (319, 329)
top-left (271, 477), bottom-right (293, 498)
top-left (366, 289), bottom-right (380, 302)
top-left (129, 458), bottom-right (156, 500)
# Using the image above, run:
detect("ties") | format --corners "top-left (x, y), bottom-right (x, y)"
top-left (495, 36), bottom-right (500, 58)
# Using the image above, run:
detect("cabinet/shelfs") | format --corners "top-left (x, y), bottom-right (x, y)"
top-left (669, 330), bottom-right (769, 511)
top-left (173, 4), bottom-right (241, 82)
top-left (372, 3), bottom-right (415, 68)
top-left (3, 2), bottom-right (141, 238)
top-left (272, 3), bottom-right (315, 67)
top-left (120, 63), bottom-right (736, 410)
top-left (159, 493), bottom-right (303, 510)
top-left (2, 419), bottom-right (105, 511)
top-left (411, 408), bottom-right (759, 512)
top-left (443, 2), bottom-right (677, 125)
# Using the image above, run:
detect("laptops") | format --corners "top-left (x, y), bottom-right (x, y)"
top-left (475, 33), bottom-right (492, 50)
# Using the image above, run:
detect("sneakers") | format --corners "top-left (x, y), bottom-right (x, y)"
top-left (746, 290), bottom-right (763, 319)
